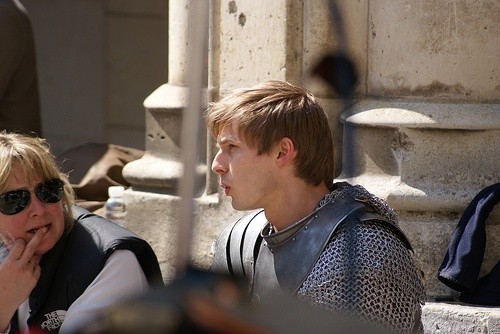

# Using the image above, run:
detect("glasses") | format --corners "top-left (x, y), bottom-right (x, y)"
top-left (0, 178), bottom-right (64, 215)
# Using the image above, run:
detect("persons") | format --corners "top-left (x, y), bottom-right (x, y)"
top-left (201, 80), bottom-right (429, 334)
top-left (0, 129), bottom-right (166, 334)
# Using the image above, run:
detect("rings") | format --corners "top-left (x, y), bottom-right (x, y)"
top-left (28, 257), bottom-right (39, 270)
